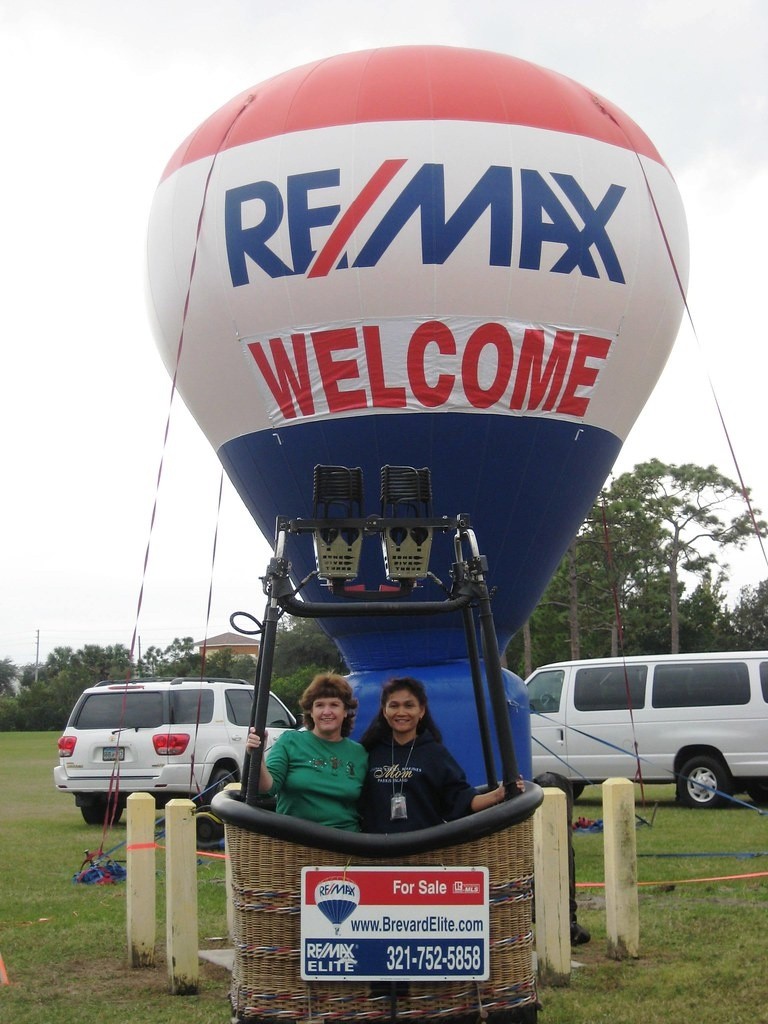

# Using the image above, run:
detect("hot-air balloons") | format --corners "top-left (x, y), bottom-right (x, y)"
top-left (140, 43), bottom-right (693, 1023)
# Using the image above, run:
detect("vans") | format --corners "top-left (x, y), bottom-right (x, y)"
top-left (523, 649), bottom-right (768, 810)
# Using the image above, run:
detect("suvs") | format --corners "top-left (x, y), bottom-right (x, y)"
top-left (53, 676), bottom-right (309, 839)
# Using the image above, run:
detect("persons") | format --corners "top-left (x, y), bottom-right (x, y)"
top-left (246, 671), bottom-right (369, 832)
top-left (359, 676), bottom-right (525, 836)
